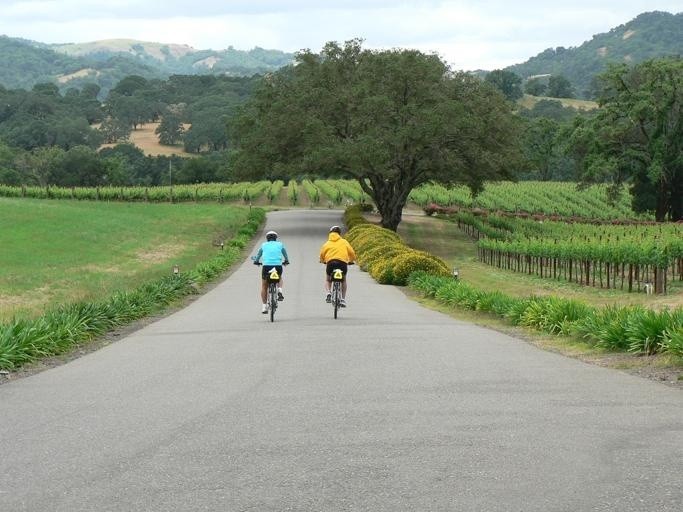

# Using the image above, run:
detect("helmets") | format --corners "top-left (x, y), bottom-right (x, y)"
top-left (330, 226), bottom-right (342, 233)
top-left (266, 231), bottom-right (278, 239)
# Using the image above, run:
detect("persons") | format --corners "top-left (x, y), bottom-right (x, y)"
top-left (254, 231), bottom-right (288, 314)
top-left (319, 226), bottom-right (355, 308)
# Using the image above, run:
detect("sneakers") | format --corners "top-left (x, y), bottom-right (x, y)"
top-left (277, 291), bottom-right (283, 301)
top-left (262, 309), bottom-right (268, 314)
top-left (326, 292), bottom-right (331, 303)
top-left (340, 299), bottom-right (347, 307)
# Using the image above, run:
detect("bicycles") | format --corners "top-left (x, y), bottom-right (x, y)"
top-left (321, 261), bottom-right (354, 319)
top-left (254, 261), bottom-right (289, 321)
